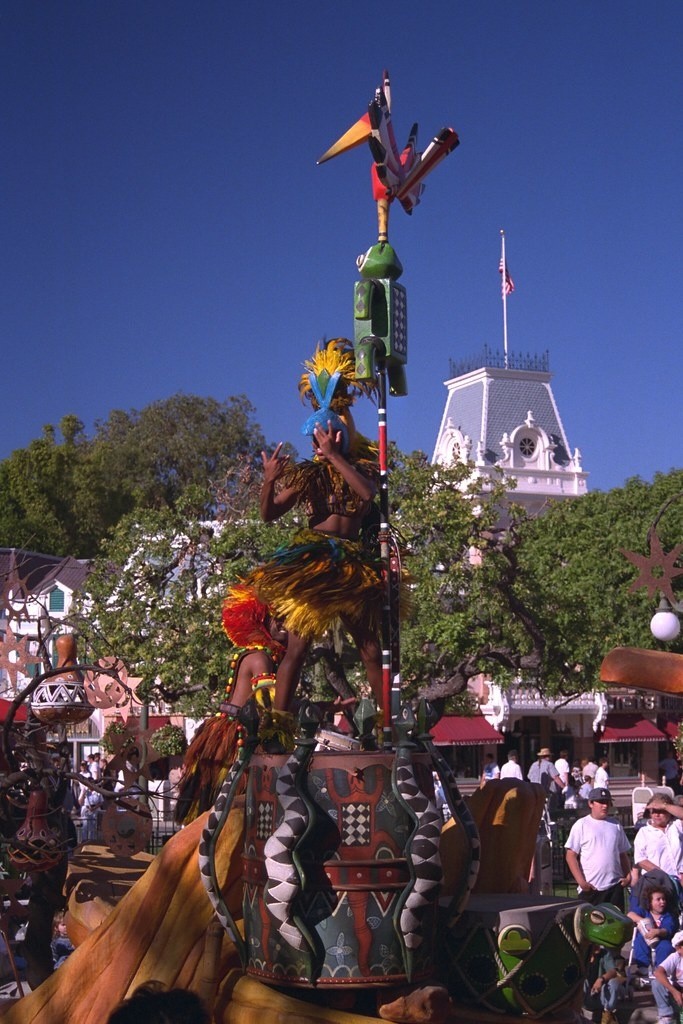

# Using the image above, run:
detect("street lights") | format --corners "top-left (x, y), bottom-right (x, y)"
top-left (649, 587), bottom-right (681, 786)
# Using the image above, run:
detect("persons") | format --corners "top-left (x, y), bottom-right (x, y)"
top-left (434, 772), bottom-right (447, 822)
top-left (258, 410), bottom-right (408, 756)
top-left (78, 753), bottom-right (109, 842)
top-left (582, 885), bottom-right (683, 1024)
top-left (481, 750), bottom-right (523, 782)
top-left (51, 910), bottom-right (76, 970)
top-left (528, 748), bottom-right (610, 815)
top-left (658, 750), bottom-right (681, 796)
top-left (630, 793), bottom-right (683, 912)
top-left (108, 980), bottom-right (212, 1024)
top-left (565, 788), bottom-right (631, 913)
top-left (174, 615), bottom-right (358, 828)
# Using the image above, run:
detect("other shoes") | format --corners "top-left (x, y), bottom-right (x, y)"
top-left (596, 1011), bottom-right (618, 1024)
top-left (654, 1016), bottom-right (676, 1024)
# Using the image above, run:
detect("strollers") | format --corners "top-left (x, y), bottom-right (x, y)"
top-left (623, 865), bottom-right (683, 1000)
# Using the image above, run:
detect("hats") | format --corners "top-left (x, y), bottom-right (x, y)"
top-left (671, 930), bottom-right (683, 948)
top-left (299, 335), bottom-right (369, 455)
top-left (219, 570), bottom-right (288, 661)
top-left (588, 788), bottom-right (616, 802)
top-left (80, 760), bottom-right (87, 765)
top-left (537, 748), bottom-right (553, 756)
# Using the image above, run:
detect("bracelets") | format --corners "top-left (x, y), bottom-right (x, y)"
top-left (663, 803), bottom-right (667, 810)
top-left (600, 976), bottom-right (605, 982)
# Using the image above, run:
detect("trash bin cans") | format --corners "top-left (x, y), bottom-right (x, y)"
top-left (632, 787), bottom-right (675, 826)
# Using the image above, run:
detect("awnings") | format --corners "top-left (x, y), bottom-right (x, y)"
top-left (429, 716), bottom-right (504, 745)
top-left (592, 718), bottom-right (680, 743)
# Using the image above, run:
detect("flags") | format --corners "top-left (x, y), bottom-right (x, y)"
top-left (500, 255), bottom-right (514, 298)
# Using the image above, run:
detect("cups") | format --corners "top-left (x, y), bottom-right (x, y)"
top-left (636, 918), bottom-right (659, 947)
